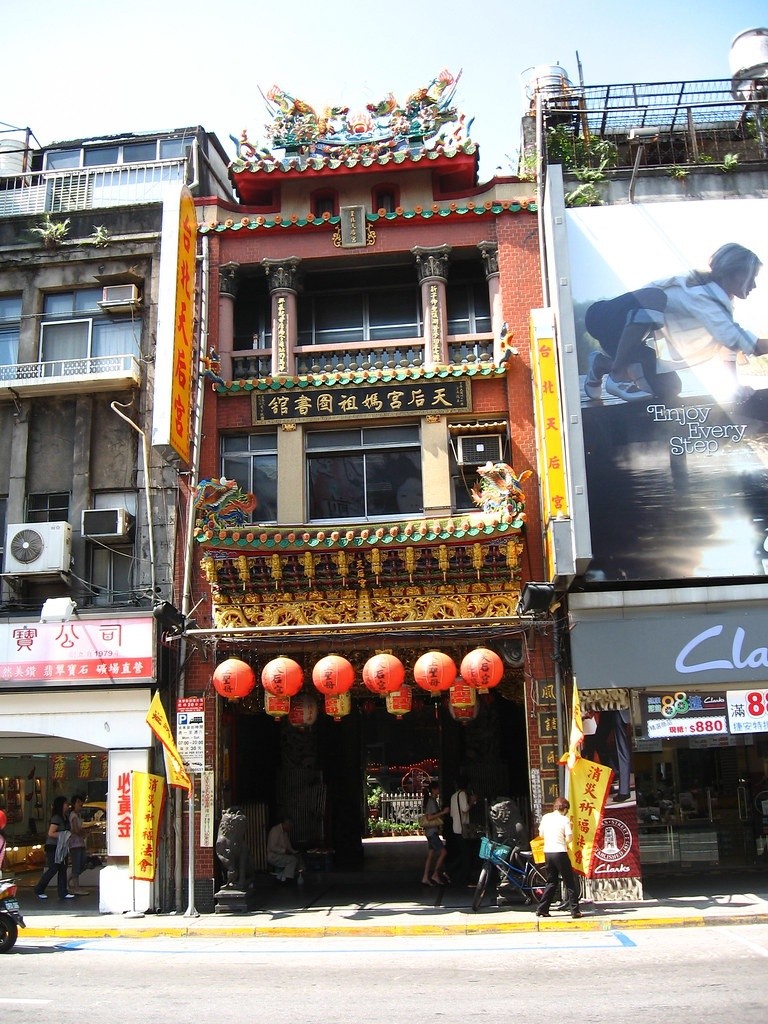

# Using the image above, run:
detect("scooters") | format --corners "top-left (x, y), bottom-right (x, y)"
top-left (0, 878), bottom-right (26, 954)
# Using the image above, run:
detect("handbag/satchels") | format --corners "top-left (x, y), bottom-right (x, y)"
top-left (419, 814), bottom-right (445, 828)
top-left (531, 837), bottom-right (545, 864)
top-left (462, 824), bottom-right (478, 839)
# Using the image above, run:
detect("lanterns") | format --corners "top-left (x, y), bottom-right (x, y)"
top-left (213, 648), bottom-right (503, 727)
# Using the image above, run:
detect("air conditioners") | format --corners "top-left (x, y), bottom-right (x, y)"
top-left (102, 285), bottom-right (140, 313)
top-left (81, 509), bottom-right (134, 545)
top-left (456, 434), bottom-right (505, 465)
top-left (1, 521), bottom-right (72, 589)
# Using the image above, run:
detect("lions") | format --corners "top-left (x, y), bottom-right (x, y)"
top-left (488, 797), bottom-right (533, 882)
top-left (215, 804), bottom-right (249, 892)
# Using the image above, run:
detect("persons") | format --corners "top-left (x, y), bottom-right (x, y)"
top-left (266, 816), bottom-right (311, 884)
top-left (422, 781), bottom-right (449, 886)
top-left (586, 710), bottom-right (631, 802)
top-left (34, 795), bottom-right (90, 899)
top-left (586, 243), bottom-right (768, 401)
top-left (450, 784), bottom-right (478, 862)
top-left (0, 811), bottom-right (7, 879)
top-left (537, 798), bottom-right (583, 918)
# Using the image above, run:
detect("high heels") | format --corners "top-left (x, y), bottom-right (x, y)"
top-left (430, 878), bottom-right (444, 886)
top-left (572, 913), bottom-right (581, 918)
top-left (536, 910), bottom-right (550, 917)
top-left (421, 880), bottom-right (434, 887)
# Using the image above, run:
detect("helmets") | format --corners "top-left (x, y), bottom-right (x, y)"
top-left (0, 811), bottom-right (7, 830)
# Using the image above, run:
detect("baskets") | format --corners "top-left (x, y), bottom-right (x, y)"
top-left (479, 837), bottom-right (511, 865)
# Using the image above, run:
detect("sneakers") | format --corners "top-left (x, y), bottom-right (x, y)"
top-left (584, 350), bottom-right (606, 399)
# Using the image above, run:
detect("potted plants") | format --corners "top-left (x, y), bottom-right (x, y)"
top-left (20, 211), bottom-right (70, 249)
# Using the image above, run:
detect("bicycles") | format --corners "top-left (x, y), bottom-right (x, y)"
top-left (471, 831), bottom-right (582, 911)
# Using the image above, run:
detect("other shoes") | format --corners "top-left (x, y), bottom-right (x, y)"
top-left (606, 375), bottom-right (653, 401)
top-left (38, 894), bottom-right (48, 898)
top-left (64, 894), bottom-right (75, 898)
top-left (614, 794), bottom-right (631, 801)
